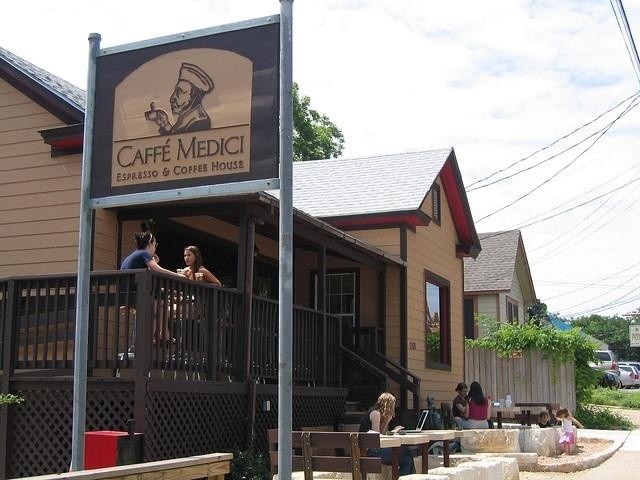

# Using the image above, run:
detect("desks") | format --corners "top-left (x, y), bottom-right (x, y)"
top-left (487, 406), bottom-right (547, 428)
top-left (361, 428), bottom-right (456, 480)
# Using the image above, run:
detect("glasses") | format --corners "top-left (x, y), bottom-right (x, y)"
top-left (155, 243), bottom-right (158, 247)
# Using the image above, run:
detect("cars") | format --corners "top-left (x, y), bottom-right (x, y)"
top-left (587, 350), bottom-right (640, 389)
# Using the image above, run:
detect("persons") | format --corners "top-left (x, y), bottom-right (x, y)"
top-left (537, 412), bottom-right (551, 428)
top-left (359, 392), bottom-right (416, 475)
top-left (121, 232), bottom-right (187, 354)
top-left (156, 246), bottom-right (222, 344)
top-left (556, 407), bottom-right (584, 456)
top-left (453, 381), bottom-right (494, 428)
top-left (144, 62), bottom-right (213, 135)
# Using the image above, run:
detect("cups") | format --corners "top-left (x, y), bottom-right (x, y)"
top-left (399, 430), bottom-right (407, 435)
top-left (386, 430), bottom-right (394, 436)
top-left (195, 273), bottom-right (204, 282)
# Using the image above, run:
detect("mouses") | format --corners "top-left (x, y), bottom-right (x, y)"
top-left (399, 432), bottom-right (406, 435)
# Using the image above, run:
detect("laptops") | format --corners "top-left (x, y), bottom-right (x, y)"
top-left (398, 409), bottom-right (430, 433)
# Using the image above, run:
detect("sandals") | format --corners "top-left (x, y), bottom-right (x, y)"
top-left (159, 337), bottom-right (177, 343)
